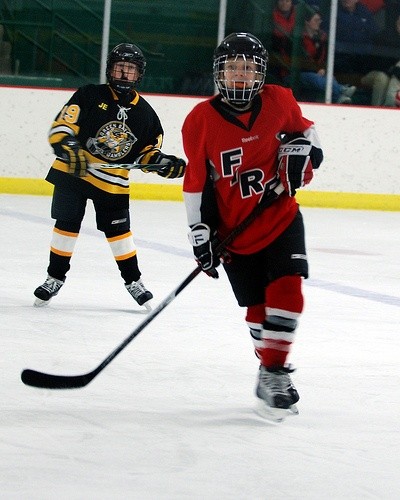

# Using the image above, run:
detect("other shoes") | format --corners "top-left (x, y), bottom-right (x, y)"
top-left (337, 95), bottom-right (352, 104)
top-left (342, 85), bottom-right (357, 97)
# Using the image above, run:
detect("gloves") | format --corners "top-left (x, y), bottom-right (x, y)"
top-left (187, 222), bottom-right (220, 280)
top-left (276, 137), bottom-right (314, 199)
top-left (58, 134), bottom-right (90, 178)
top-left (147, 151), bottom-right (186, 181)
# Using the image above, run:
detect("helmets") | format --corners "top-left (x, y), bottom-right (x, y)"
top-left (105, 42), bottom-right (147, 94)
top-left (212, 32), bottom-right (268, 105)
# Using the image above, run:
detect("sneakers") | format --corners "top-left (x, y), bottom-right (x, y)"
top-left (124, 276), bottom-right (154, 312)
top-left (254, 364), bottom-right (301, 424)
top-left (33, 275), bottom-right (67, 308)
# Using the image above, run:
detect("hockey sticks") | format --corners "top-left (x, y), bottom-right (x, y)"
top-left (20, 179), bottom-right (287, 390)
top-left (87, 163), bottom-right (169, 169)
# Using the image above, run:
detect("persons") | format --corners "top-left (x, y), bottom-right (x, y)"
top-left (183, 32), bottom-right (324, 409)
top-left (273, 0), bottom-right (400, 106)
top-left (33, 43), bottom-right (186, 305)
